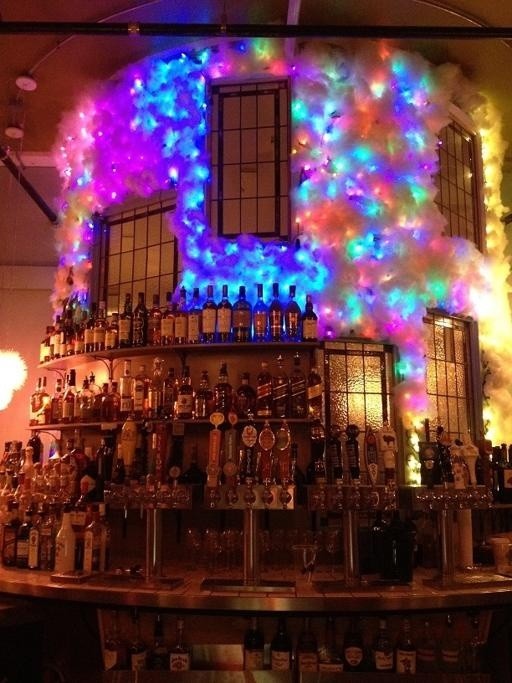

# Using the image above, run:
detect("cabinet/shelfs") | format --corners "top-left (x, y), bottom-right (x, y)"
top-left (27, 338), bottom-right (322, 429)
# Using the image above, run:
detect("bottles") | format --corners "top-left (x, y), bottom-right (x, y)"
top-left (102, 607), bottom-right (195, 672)
top-left (27, 350), bottom-right (321, 426)
top-left (1, 424), bottom-right (511, 581)
top-left (241, 611), bottom-right (486, 673)
top-left (37, 281), bottom-right (316, 362)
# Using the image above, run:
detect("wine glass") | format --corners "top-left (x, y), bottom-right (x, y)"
top-left (291, 544), bottom-right (319, 592)
